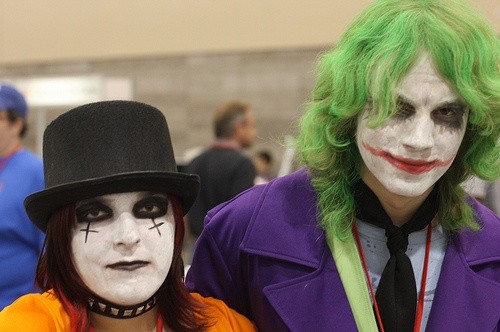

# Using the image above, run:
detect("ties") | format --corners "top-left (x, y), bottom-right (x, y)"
top-left (346, 169), bottom-right (443, 332)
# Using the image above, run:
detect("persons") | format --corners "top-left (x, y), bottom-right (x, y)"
top-left (0, 101), bottom-right (255, 332)
top-left (183, 103), bottom-right (260, 265)
top-left (0, 85), bottom-right (50, 311)
top-left (185, 0), bottom-right (500, 332)
top-left (250, 152), bottom-right (272, 187)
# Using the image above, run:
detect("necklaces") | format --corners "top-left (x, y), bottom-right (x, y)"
top-left (350, 220), bottom-right (432, 332)
top-left (85, 292), bottom-right (164, 320)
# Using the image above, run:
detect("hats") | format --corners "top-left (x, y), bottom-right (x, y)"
top-left (24, 100), bottom-right (200, 236)
top-left (0, 80), bottom-right (28, 118)
